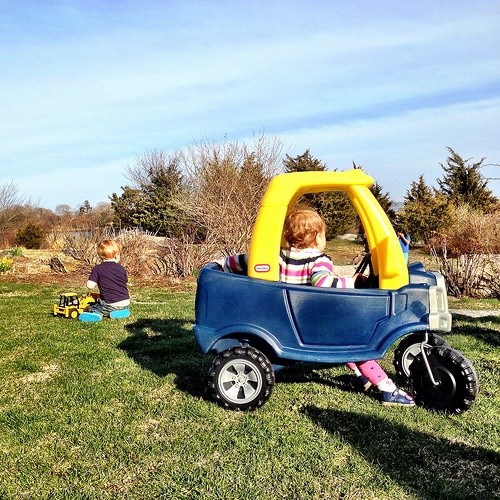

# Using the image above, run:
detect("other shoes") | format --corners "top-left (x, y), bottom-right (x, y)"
top-left (379, 389), bottom-right (415, 408)
top-left (357, 375), bottom-right (373, 392)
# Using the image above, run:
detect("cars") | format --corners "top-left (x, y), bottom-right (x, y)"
top-left (191, 168), bottom-right (480, 416)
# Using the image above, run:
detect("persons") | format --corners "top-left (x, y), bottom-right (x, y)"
top-left (83, 239), bottom-right (131, 317)
top-left (210, 207), bottom-right (418, 407)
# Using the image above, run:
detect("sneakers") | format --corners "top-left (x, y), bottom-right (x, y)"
top-left (109, 309), bottom-right (130, 318)
top-left (78, 313), bottom-right (102, 322)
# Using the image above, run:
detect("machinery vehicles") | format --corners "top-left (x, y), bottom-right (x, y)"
top-left (52, 291), bottom-right (95, 319)
